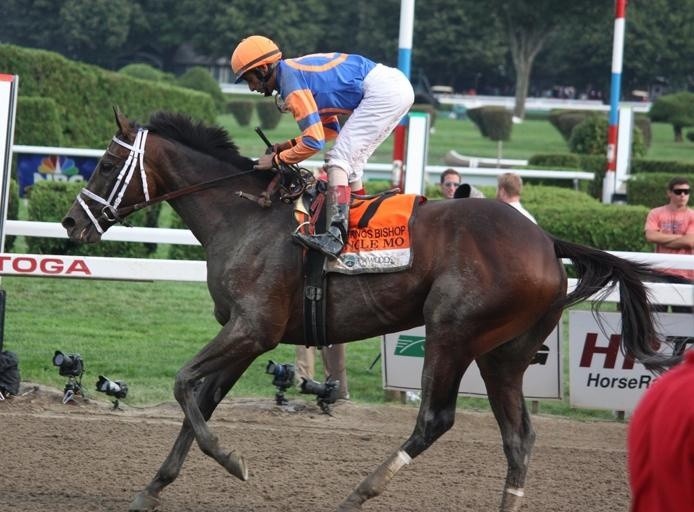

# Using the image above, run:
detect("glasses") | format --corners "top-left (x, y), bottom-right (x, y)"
top-left (442, 182), bottom-right (461, 187)
top-left (672, 188), bottom-right (691, 195)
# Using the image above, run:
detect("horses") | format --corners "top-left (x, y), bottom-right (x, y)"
top-left (60, 103), bottom-right (689, 512)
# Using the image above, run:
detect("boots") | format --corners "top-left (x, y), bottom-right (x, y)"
top-left (298, 186), bottom-right (350, 257)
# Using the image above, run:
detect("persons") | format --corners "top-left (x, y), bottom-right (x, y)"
top-left (643, 176), bottom-right (693, 314)
top-left (456, 73), bottom-right (637, 107)
top-left (437, 168), bottom-right (461, 198)
top-left (494, 172), bottom-right (538, 227)
top-left (319, 343), bottom-right (349, 404)
top-left (231, 34), bottom-right (415, 257)
top-left (293, 345), bottom-right (314, 385)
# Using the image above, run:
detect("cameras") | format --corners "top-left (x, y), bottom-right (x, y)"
top-left (95, 375), bottom-right (128, 398)
top-left (53, 349), bottom-right (82, 376)
top-left (264, 360), bottom-right (295, 387)
top-left (300, 376), bottom-right (340, 403)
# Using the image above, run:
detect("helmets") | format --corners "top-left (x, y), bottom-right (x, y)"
top-left (231, 35), bottom-right (283, 84)
